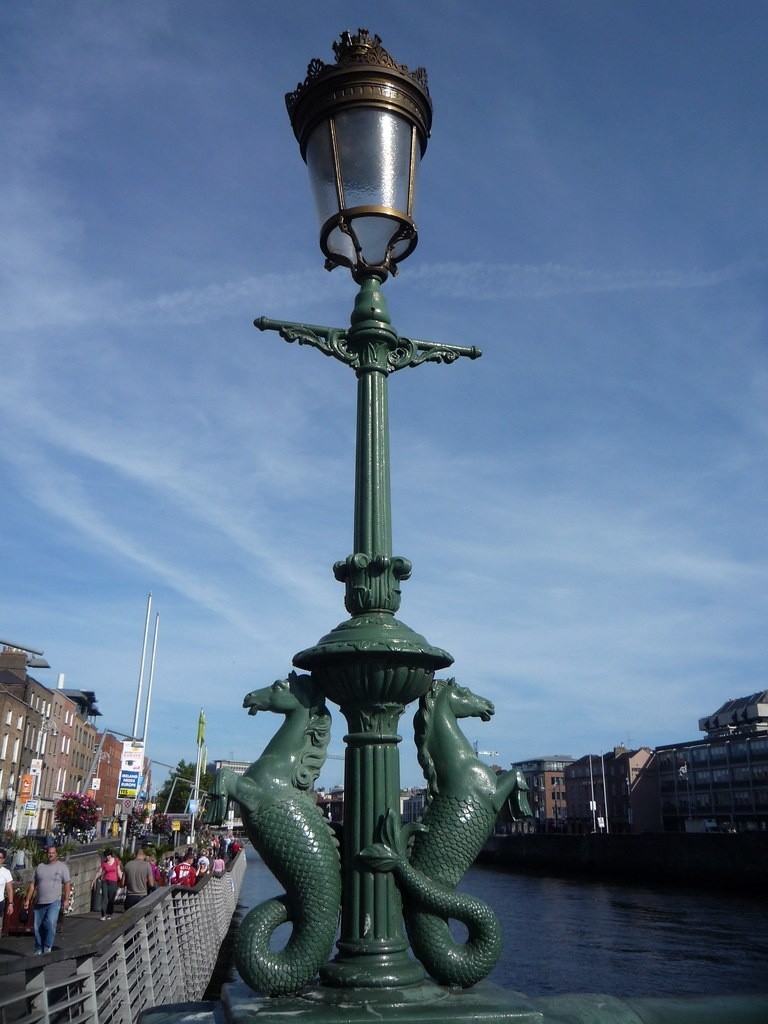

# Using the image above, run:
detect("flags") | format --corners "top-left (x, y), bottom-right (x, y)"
top-left (201, 751), bottom-right (207, 771)
top-left (197, 712), bottom-right (206, 747)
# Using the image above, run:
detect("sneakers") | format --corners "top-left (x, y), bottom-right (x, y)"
top-left (100, 916), bottom-right (105, 920)
top-left (33, 948), bottom-right (42, 955)
top-left (107, 914), bottom-right (112, 920)
top-left (44, 947), bottom-right (51, 954)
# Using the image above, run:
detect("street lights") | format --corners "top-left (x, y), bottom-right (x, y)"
top-left (163, 776), bottom-right (195, 815)
top-left (185, 785), bottom-right (212, 813)
top-left (81, 729), bottom-right (146, 795)
top-left (212, 26), bottom-right (533, 995)
top-left (135, 760), bottom-right (178, 810)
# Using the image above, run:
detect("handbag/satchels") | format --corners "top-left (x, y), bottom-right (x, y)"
top-left (115, 886), bottom-right (127, 901)
top-left (117, 859), bottom-right (122, 883)
top-left (19, 909), bottom-right (29, 923)
top-left (91, 879), bottom-right (102, 911)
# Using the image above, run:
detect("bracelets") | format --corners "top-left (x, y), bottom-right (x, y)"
top-left (9, 902), bottom-right (13, 905)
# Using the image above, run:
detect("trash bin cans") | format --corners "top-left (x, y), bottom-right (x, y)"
top-left (91, 888), bottom-right (102, 911)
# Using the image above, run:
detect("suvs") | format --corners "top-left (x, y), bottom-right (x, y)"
top-left (19, 828), bottom-right (55, 850)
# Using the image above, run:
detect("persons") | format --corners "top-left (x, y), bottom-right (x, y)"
top-left (90, 850), bottom-right (123, 922)
top-left (0, 848), bottom-right (14, 938)
top-left (23, 846), bottom-right (71, 956)
top-left (145, 846), bottom-right (210, 915)
top-left (121, 848), bottom-right (155, 914)
top-left (206, 833), bottom-right (241, 875)
